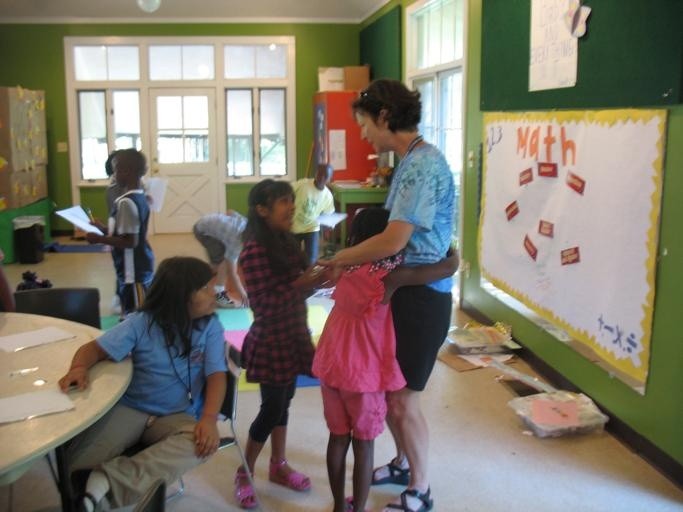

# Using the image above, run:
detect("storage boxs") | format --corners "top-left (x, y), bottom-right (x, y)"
top-left (318, 66), bottom-right (369, 91)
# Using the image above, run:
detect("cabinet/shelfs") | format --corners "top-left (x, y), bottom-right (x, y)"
top-left (313, 92), bottom-right (378, 241)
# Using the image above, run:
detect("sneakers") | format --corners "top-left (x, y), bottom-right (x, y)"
top-left (71, 469), bottom-right (96, 512)
top-left (215, 291), bottom-right (234, 308)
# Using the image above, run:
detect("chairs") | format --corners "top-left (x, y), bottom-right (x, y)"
top-left (13, 288), bottom-right (100, 329)
top-left (123, 341), bottom-right (264, 511)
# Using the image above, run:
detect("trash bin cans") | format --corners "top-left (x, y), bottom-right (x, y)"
top-left (12, 216), bottom-right (46, 263)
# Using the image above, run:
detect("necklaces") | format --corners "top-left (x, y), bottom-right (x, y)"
top-left (164, 324), bottom-right (194, 407)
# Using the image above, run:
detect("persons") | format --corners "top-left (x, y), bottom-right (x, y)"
top-left (234, 179), bottom-right (323, 510)
top-left (287, 163), bottom-right (336, 264)
top-left (316, 80), bottom-right (455, 512)
top-left (311, 207), bottom-right (459, 511)
top-left (84, 150), bottom-right (154, 321)
top-left (57, 257), bottom-right (230, 512)
top-left (192, 209), bottom-right (251, 308)
top-left (105, 150), bottom-right (153, 321)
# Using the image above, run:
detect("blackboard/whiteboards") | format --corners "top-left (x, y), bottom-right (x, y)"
top-left (479, 108), bottom-right (671, 398)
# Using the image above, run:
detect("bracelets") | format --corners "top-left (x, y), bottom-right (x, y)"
top-left (202, 413), bottom-right (218, 422)
top-left (68, 364), bottom-right (88, 371)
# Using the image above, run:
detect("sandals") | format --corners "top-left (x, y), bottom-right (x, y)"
top-left (371, 458), bottom-right (409, 485)
top-left (234, 464), bottom-right (257, 508)
top-left (270, 459), bottom-right (311, 491)
top-left (386, 485), bottom-right (432, 512)
top-left (345, 496), bottom-right (353, 512)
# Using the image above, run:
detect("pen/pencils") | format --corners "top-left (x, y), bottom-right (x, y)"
top-left (86, 208), bottom-right (95, 223)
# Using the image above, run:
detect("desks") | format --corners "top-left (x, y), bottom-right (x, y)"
top-left (0, 313), bottom-right (134, 512)
top-left (328, 183), bottom-right (388, 249)
